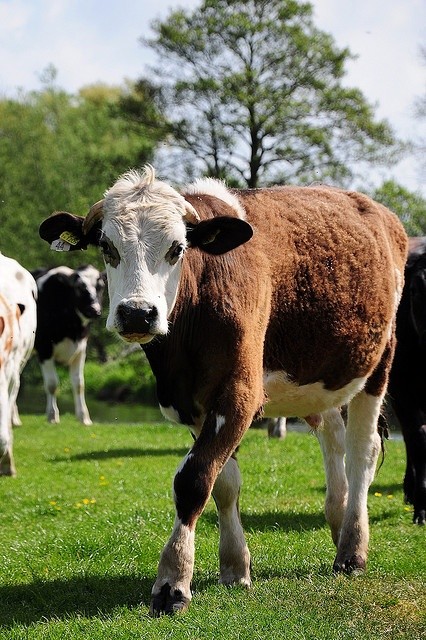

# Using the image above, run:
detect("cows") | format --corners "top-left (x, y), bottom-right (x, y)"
top-left (29, 265), bottom-right (106, 425)
top-left (37, 163), bottom-right (410, 620)
top-left (0, 253), bottom-right (38, 478)
top-left (386, 237), bottom-right (424, 524)
